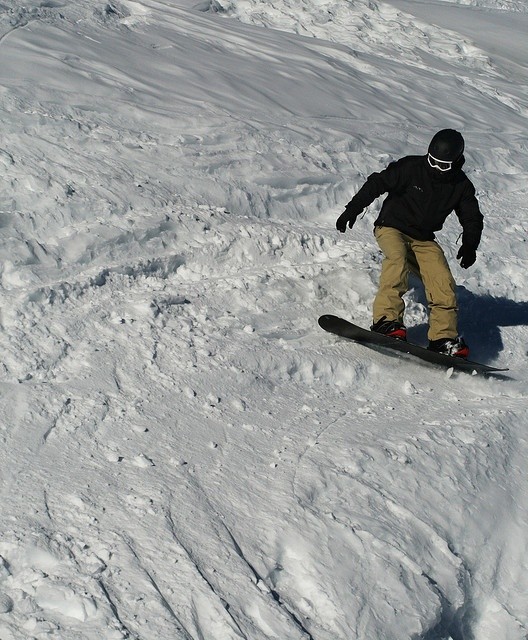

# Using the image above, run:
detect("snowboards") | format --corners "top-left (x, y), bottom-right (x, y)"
top-left (319, 314), bottom-right (509, 374)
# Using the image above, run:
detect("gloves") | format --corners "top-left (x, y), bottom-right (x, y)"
top-left (456, 243), bottom-right (477, 269)
top-left (336, 208), bottom-right (358, 233)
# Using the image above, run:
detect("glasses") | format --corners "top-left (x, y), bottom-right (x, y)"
top-left (427, 152), bottom-right (455, 172)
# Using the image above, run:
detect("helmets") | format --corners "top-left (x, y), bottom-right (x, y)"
top-left (426, 129), bottom-right (466, 174)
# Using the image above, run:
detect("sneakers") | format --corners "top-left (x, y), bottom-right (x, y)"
top-left (428, 337), bottom-right (468, 356)
top-left (370, 319), bottom-right (406, 340)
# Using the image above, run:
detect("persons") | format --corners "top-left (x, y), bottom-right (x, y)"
top-left (335, 128), bottom-right (484, 355)
